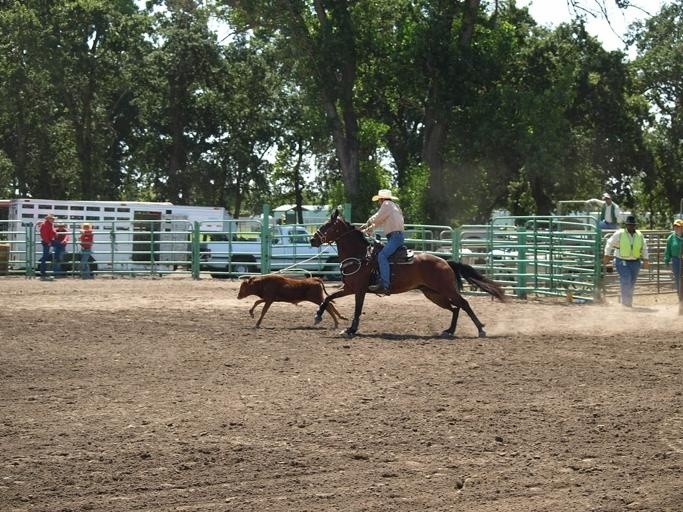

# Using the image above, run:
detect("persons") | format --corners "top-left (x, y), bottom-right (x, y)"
top-left (602, 216), bottom-right (649, 311)
top-left (663, 218), bottom-right (683, 314)
top-left (359, 189), bottom-right (404, 294)
top-left (38, 212), bottom-right (57, 280)
top-left (600, 193), bottom-right (620, 228)
top-left (50, 221), bottom-right (69, 271)
top-left (78, 222), bottom-right (94, 279)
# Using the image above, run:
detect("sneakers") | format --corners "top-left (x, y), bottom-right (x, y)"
top-left (368, 279), bottom-right (390, 296)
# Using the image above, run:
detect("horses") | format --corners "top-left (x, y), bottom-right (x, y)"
top-left (310, 207), bottom-right (507, 338)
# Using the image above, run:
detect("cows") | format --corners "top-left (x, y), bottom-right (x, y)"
top-left (237, 272), bottom-right (348, 329)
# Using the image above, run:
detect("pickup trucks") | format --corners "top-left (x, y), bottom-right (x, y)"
top-left (192, 224), bottom-right (344, 280)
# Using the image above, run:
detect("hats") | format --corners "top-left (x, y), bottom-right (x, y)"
top-left (372, 189), bottom-right (399, 202)
top-left (44, 214), bottom-right (57, 220)
top-left (673, 219), bottom-right (683, 227)
top-left (80, 223), bottom-right (92, 233)
top-left (623, 216), bottom-right (640, 225)
